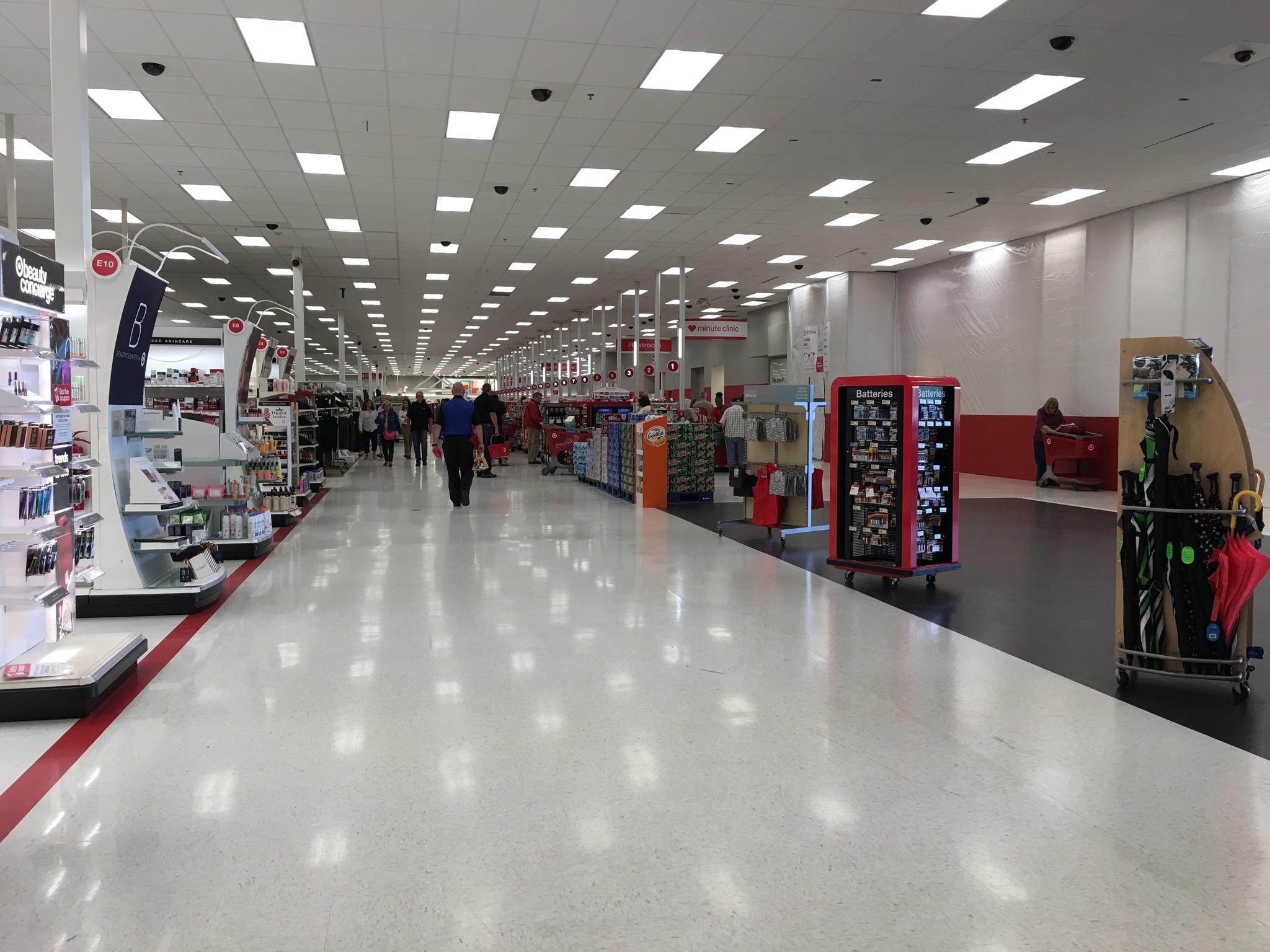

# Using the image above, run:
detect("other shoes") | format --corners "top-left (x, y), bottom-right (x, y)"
top-left (377, 453), bottom-right (383, 458)
top-left (404, 455), bottom-right (409, 459)
top-left (416, 462), bottom-right (421, 466)
top-left (423, 460), bottom-right (427, 466)
top-left (373, 455), bottom-right (377, 459)
top-left (389, 462), bottom-right (393, 467)
top-left (530, 460), bottom-right (541, 464)
top-left (364, 454), bottom-right (368, 459)
top-left (528, 462), bottom-right (530, 463)
top-left (383, 462), bottom-right (388, 466)
top-left (409, 456), bottom-right (412, 459)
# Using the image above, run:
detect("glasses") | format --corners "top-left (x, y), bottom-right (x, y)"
top-left (1048, 406), bottom-right (1057, 410)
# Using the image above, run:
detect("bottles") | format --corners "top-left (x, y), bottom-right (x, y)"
top-left (215, 531), bottom-right (222, 538)
top-left (222, 513), bottom-right (230, 539)
top-left (278, 441), bottom-right (286, 445)
top-left (242, 509), bottom-right (248, 534)
top-left (257, 463), bottom-right (261, 471)
top-left (247, 505), bottom-right (272, 539)
top-left (253, 462), bottom-right (257, 470)
top-left (270, 462), bottom-right (275, 471)
top-left (221, 507), bottom-right (249, 531)
top-left (265, 462), bottom-right (270, 470)
top-left (251, 466), bottom-right (257, 480)
top-left (261, 462), bottom-right (266, 470)
top-left (236, 513), bottom-right (243, 538)
top-left (230, 512), bottom-right (236, 538)
top-left (233, 480), bottom-right (238, 499)
top-left (243, 435), bottom-right (274, 453)
top-left (245, 480), bottom-right (250, 499)
top-left (274, 450), bottom-right (286, 455)
top-left (273, 378), bottom-right (295, 393)
top-left (258, 466), bottom-right (265, 480)
top-left (311, 467), bottom-right (324, 480)
top-left (248, 463), bottom-right (253, 475)
top-left (153, 444), bottom-right (169, 459)
top-left (275, 462), bottom-right (279, 471)
top-left (244, 408), bottom-right (263, 417)
top-left (263, 431), bottom-right (286, 436)
top-left (265, 466), bottom-right (271, 480)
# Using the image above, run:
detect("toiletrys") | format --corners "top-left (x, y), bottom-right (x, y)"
top-left (246, 434), bottom-right (275, 453)
top-left (226, 477), bottom-right (231, 498)
top-left (245, 479), bottom-right (249, 499)
top-left (238, 478), bottom-right (244, 498)
top-left (232, 479), bottom-right (238, 499)
top-left (263, 486), bottom-right (297, 511)
top-left (144, 367), bottom-right (224, 384)
top-left (249, 457), bottom-right (281, 480)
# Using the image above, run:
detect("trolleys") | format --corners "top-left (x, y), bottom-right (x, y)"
top-left (540, 421), bottom-right (597, 476)
top-left (1037, 423), bottom-right (1103, 492)
top-left (502, 416), bottom-right (519, 452)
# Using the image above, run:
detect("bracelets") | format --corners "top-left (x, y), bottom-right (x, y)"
top-left (479, 444), bottom-right (484, 446)
top-left (432, 444), bottom-right (437, 446)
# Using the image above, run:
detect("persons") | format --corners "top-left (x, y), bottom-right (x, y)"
top-left (491, 395), bottom-right (511, 466)
top-left (333, 382), bottom-right (381, 408)
top-left (374, 400), bottom-right (402, 467)
top-left (637, 395), bottom-right (657, 414)
top-left (359, 399), bottom-right (379, 460)
top-left (433, 382), bottom-right (485, 506)
top-left (405, 391), bottom-right (432, 466)
top-left (719, 398), bottom-right (745, 474)
top-left (1034, 397), bottom-right (1066, 486)
top-left (434, 398), bottom-right (450, 440)
top-left (711, 392), bottom-right (730, 426)
top-left (693, 391), bottom-right (714, 421)
top-left (521, 396), bottom-right (526, 400)
top-left (399, 398), bottom-right (413, 459)
top-left (376, 395), bottom-right (393, 458)
top-left (522, 392), bottom-right (546, 464)
top-left (474, 383), bottom-right (499, 477)
top-left (691, 399), bottom-right (698, 405)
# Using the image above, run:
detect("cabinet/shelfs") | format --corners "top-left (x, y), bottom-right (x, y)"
top-left (0, 239), bottom-right (363, 724)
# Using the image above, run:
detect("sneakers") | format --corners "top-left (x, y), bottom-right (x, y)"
top-left (454, 502), bottom-right (461, 507)
top-left (1047, 479), bottom-right (1060, 486)
top-left (477, 473), bottom-right (482, 477)
top-left (482, 472), bottom-right (496, 478)
top-left (1036, 482), bottom-right (1047, 487)
top-left (460, 490), bottom-right (470, 506)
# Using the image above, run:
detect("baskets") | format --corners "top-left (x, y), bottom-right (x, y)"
top-left (488, 434), bottom-right (509, 459)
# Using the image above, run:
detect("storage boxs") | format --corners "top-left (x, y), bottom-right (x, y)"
top-left (572, 413), bottom-right (715, 494)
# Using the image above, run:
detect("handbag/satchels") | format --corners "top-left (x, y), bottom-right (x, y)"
top-left (384, 431), bottom-right (395, 440)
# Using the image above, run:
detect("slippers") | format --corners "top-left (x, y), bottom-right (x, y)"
top-left (502, 463), bottom-right (510, 466)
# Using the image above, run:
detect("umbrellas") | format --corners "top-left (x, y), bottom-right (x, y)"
top-left (1117, 393), bottom-right (1270, 675)
